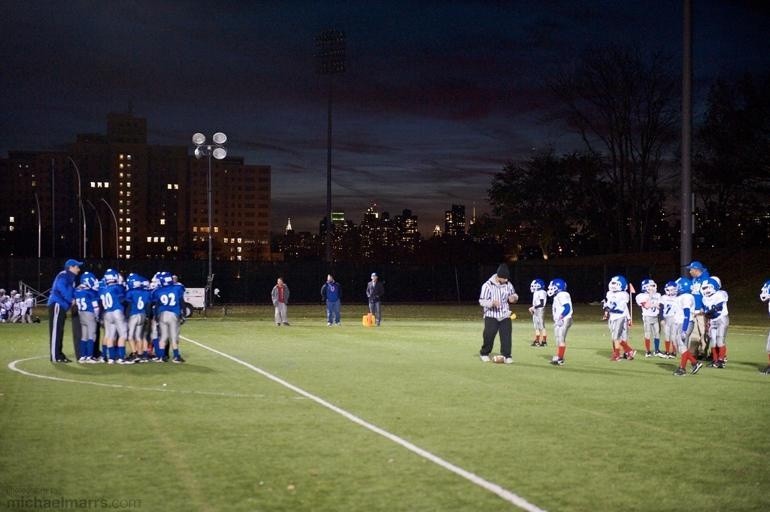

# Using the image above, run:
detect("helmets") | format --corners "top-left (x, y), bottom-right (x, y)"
top-left (530, 278), bottom-right (545, 293)
top-left (80, 268), bottom-right (174, 290)
top-left (760, 281), bottom-right (770, 302)
top-left (547, 278), bottom-right (567, 297)
top-left (0, 288), bottom-right (33, 301)
top-left (641, 261), bottom-right (722, 297)
top-left (608, 275), bottom-right (628, 291)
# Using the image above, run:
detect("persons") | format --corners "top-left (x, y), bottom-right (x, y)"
top-left (547, 278), bottom-right (575, 366)
top-left (477, 263), bottom-right (519, 365)
top-left (320, 273), bottom-right (345, 328)
top-left (0, 287), bottom-right (41, 325)
top-left (365, 271), bottom-right (386, 326)
top-left (600, 261), bottom-right (730, 377)
top-left (528, 278), bottom-right (549, 347)
top-left (759, 276), bottom-right (770, 376)
top-left (271, 276), bottom-right (291, 328)
top-left (46, 258), bottom-right (186, 365)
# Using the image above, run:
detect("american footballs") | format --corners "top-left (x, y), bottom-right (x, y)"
top-left (492, 354), bottom-right (505, 362)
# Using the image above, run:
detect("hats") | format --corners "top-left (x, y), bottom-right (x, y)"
top-left (497, 264), bottom-right (510, 275)
top-left (64, 259), bottom-right (83, 270)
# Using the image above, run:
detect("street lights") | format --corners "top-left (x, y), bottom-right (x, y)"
top-left (192, 130), bottom-right (229, 307)
top-left (311, 25), bottom-right (347, 278)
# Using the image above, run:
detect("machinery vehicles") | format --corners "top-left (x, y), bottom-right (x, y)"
top-left (183, 288), bottom-right (207, 317)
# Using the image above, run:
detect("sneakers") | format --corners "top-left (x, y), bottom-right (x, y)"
top-left (540, 341), bottom-right (547, 346)
top-left (282, 322), bottom-right (290, 326)
top-left (504, 357), bottom-right (513, 363)
top-left (327, 322), bottom-right (332, 327)
top-left (57, 352), bottom-right (185, 364)
top-left (690, 361), bottom-right (703, 374)
top-left (481, 355), bottom-right (490, 362)
top-left (532, 341), bottom-right (540, 346)
top-left (612, 349), bottom-right (728, 369)
top-left (759, 366), bottom-right (770, 374)
top-left (674, 368), bottom-right (686, 376)
top-left (551, 358), bottom-right (565, 366)
